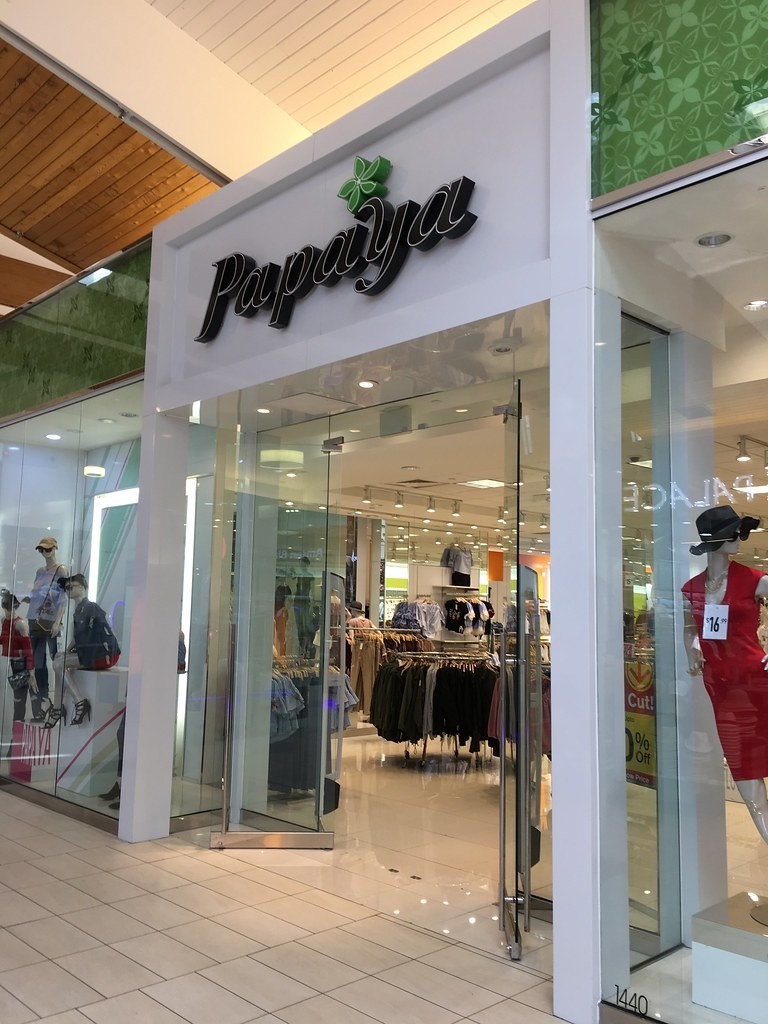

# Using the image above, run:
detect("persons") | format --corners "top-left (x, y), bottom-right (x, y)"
top-left (99, 631), bottom-right (186, 809)
top-left (330, 589), bottom-right (352, 665)
top-left (26, 536), bottom-right (69, 722)
top-left (349, 601), bottom-right (382, 651)
top-left (272, 585), bottom-right (292, 656)
top-left (0, 594), bottom-right (39, 758)
top-left (680, 504), bottom-right (768, 844)
top-left (40, 574), bottom-right (121, 729)
top-left (290, 557), bottom-right (315, 658)
top-left (449, 543), bottom-right (474, 585)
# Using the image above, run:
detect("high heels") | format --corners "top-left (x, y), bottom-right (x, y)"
top-left (98, 781), bottom-right (123, 801)
top-left (30, 697), bottom-right (53, 722)
top-left (108, 802), bottom-right (120, 810)
top-left (41, 706), bottom-right (67, 729)
top-left (71, 697), bottom-right (91, 724)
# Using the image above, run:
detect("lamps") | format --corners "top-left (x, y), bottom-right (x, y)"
top-left (497, 506), bottom-right (505, 524)
top-left (395, 491), bottom-right (405, 507)
top-left (519, 511), bottom-right (526, 527)
top-left (539, 515), bottom-right (548, 528)
top-left (361, 483), bottom-right (371, 503)
top-left (451, 500), bottom-right (462, 518)
top-left (426, 497), bottom-right (437, 513)
top-left (390, 535), bottom-right (536, 563)
top-left (735, 435), bottom-right (751, 462)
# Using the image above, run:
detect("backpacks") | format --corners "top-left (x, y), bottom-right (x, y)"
top-left (87, 602), bottom-right (121, 669)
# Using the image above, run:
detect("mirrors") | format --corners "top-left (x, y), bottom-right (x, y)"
top-left (384, 519), bottom-right (410, 628)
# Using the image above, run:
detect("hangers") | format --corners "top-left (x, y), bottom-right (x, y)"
top-left (397, 651), bottom-right (498, 676)
top-left (273, 654), bottom-right (342, 679)
top-left (632, 622), bottom-right (655, 664)
top-left (521, 600), bottom-right (549, 615)
top-left (506, 632), bottom-right (550, 648)
top-left (352, 627), bottom-right (426, 642)
top-left (453, 592), bottom-right (481, 604)
top-left (413, 593), bottom-right (433, 604)
top-left (506, 657), bottom-right (548, 683)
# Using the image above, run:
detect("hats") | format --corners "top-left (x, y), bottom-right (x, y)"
top-left (57, 574), bottom-right (88, 590)
top-left (36, 537), bottom-right (59, 550)
top-left (348, 601), bottom-right (365, 614)
top-left (689, 506), bottom-right (761, 554)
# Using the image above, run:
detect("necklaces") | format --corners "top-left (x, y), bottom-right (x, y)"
top-left (705, 575), bottom-right (725, 593)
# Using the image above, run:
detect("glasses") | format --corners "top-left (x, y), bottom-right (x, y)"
top-left (64, 584), bottom-right (81, 592)
top-left (38, 548), bottom-right (53, 553)
top-left (6, 604), bottom-right (19, 611)
top-left (727, 527), bottom-right (750, 542)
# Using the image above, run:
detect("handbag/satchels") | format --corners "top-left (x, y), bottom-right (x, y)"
top-left (7, 669), bottom-right (30, 689)
top-left (33, 619), bottom-right (63, 637)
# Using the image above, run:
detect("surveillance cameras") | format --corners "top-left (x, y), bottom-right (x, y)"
top-left (630, 457), bottom-right (639, 461)
